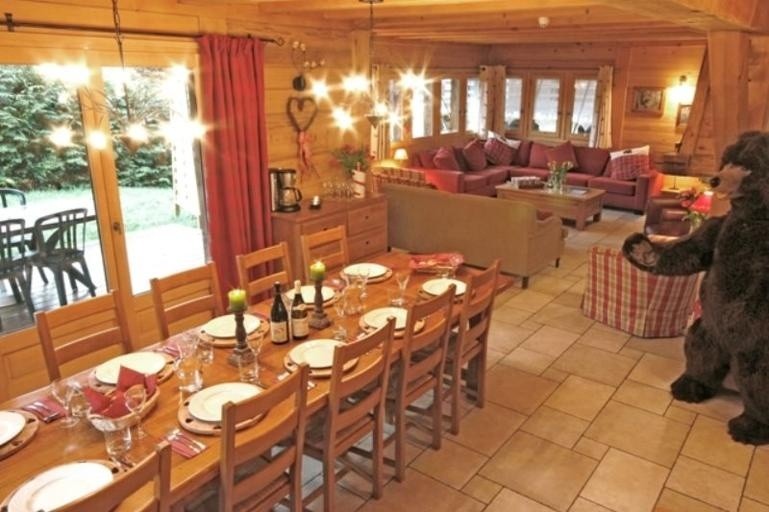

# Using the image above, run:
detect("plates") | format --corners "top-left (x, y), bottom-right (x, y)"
top-left (203, 315), bottom-right (261, 338)
top-left (0, 409), bottom-right (27, 447)
top-left (95, 351), bottom-right (166, 385)
top-left (343, 263), bottom-right (387, 278)
top-left (4, 462), bottom-right (114, 512)
top-left (185, 383), bottom-right (264, 423)
top-left (421, 278), bottom-right (468, 295)
top-left (363, 307), bottom-right (408, 330)
top-left (284, 285), bottom-right (335, 304)
top-left (288, 339), bottom-right (346, 368)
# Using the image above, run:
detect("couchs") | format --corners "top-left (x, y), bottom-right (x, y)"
top-left (375, 181), bottom-right (567, 290)
top-left (408, 139), bottom-right (664, 215)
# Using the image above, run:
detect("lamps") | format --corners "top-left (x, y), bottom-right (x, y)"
top-left (393, 149), bottom-right (409, 167)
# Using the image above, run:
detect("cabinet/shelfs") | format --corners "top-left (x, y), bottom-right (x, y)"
top-left (271, 192), bottom-right (388, 288)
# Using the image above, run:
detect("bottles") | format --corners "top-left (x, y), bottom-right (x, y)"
top-left (292, 280), bottom-right (309, 339)
top-left (270, 282), bottom-right (288, 345)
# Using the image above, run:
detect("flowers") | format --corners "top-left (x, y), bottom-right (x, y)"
top-left (547, 160), bottom-right (573, 175)
top-left (326, 142), bottom-right (375, 179)
top-left (676, 184), bottom-right (710, 226)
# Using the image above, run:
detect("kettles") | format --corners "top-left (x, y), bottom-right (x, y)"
top-left (273, 168), bottom-right (301, 212)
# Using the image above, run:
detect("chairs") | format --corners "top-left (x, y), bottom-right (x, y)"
top-left (0, 188), bottom-right (51, 285)
top-left (412, 257), bottom-right (502, 435)
top-left (348, 283), bottom-right (456, 481)
top-left (236, 241), bottom-right (295, 309)
top-left (190, 361), bottom-right (311, 512)
top-left (643, 198), bottom-right (692, 235)
top-left (279, 317), bottom-right (398, 512)
top-left (583, 235), bottom-right (704, 339)
top-left (23, 208), bottom-right (97, 307)
top-left (0, 219), bottom-right (37, 330)
top-left (298, 224), bottom-right (351, 285)
top-left (50, 438), bottom-right (176, 512)
top-left (149, 259), bottom-right (224, 340)
top-left (33, 287), bottom-right (136, 382)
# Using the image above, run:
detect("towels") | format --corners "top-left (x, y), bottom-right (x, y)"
top-left (18, 397), bottom-right (72, 423)
top-left (158, 427), bottom-right (209, 460)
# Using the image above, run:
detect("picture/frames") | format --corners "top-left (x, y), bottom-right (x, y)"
top-left (675, 103), bottom-right (694, 132)
top-left (632, 86), bottom-right (666, 118)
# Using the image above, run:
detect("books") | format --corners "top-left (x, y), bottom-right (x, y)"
top-left (512, 176), bottom-right (543, 189)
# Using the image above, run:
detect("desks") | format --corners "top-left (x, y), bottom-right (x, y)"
top-left (0, 196), bottom-right (96, 239)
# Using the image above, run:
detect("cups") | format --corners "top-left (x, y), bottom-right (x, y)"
top-left (449, 252), bottom-right (460, 279)
top-left (51, 374), bottom-right (79, 429)
top-left (245, 332), bottom-right (265, 357)
top-left (239, 352), bottom-right (258, 384)
top-left (177, 356), bottom-right (203, 394)
top-left (104, 419), bottom-right (131, 472)
top-left (394, 271), bottom-right (410, 304)
top-left (338, 270), bottom-right (353, 289)
top-left (174, 330), bottom-right (201, 358)
top-left (320, 178), bottom-right (353, 200)
top-left (197, 334), bottom-right (213, 366)
top-left (332, 294), bottom-right (347, 319)
top-left (124, 382), bottom-right (147, 440)
top-left (357, 265), bottom-right (370, 299)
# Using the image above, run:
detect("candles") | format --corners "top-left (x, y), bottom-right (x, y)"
top-left (309, 262), bottom-right (326, 280)
top-left (228, 289), bottom-right (248, 309)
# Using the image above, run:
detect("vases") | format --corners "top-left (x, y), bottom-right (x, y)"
top-left (546, 175), bottom-right (569, 194)
top-left (686, 225), bottom-right (698, 236)
top-left (350, 169), bottom-right (366, 198)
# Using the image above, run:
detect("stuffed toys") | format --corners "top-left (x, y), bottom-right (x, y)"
top-left (620, 132), bottom-right (768, 445)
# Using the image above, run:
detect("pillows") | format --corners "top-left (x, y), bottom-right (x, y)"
top-left (544, 138), bottom-right (580, 172)
top-left (434, 143), bottom-right (461, 172)
top-left (460, 137), bottom-right (488, 171)
top-left (609, 144), bottom-right (651, 183)
top-left (484, 130), bottom-right (521, 167)
